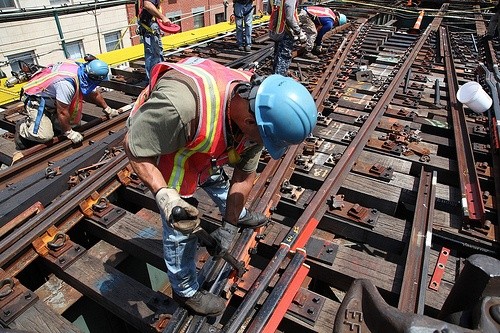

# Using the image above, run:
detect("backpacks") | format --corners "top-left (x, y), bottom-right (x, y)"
top-left (269, 9), bottom-right (286, 40)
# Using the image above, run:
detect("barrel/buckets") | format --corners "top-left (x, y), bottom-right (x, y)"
top-left (456, 81), bottom-right (492, 113)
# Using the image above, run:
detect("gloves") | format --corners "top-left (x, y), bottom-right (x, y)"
top-left (64, 129), bottom-right (83, 143)
top-left (153, 187), bottom-right (202, 234)
top-left (102, 106), bottom-right (119, 119)
top-left (210, 219), bottom-right (238, 261)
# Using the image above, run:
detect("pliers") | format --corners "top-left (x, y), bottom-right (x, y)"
top-left (172, 207), bottom-right (246, 278)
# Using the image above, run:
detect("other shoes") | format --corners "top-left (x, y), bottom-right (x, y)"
top-left (238, 46), bottom-right (244, 50)
top-left (246, 46), bottom-right (250, 51)
top-left (313, 46), bottom-right (328, 54)
top-left (304, 53), bottom-right (317, 59)
top-left (15, 122), bottom-right (26, 150)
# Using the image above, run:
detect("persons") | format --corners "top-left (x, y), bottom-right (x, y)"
top-left (123, 57), bottom-right (316, 316)
top-left (298, 7), bottom-right (346, 59)
top-left (14, 59), bottom-right (119, 150)
top-left (135, 0), bottom-right (172, 81)
top-left (267, 0), bottom-right (306, 77)
top-left (222, 0), bottom-right (255, 52)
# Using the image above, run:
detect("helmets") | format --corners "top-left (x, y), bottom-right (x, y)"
top-left (86, 60), bottom-right (109, 81)
top-left (238, 71), bottom-right (317, 160)
top-left (338, 11), bottom-right (346, 26)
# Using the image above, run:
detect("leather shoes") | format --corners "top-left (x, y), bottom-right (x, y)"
top-left (222, 211), bottom-right (268, 229)
top-left (172, 287), bottom-right (226, 317)
